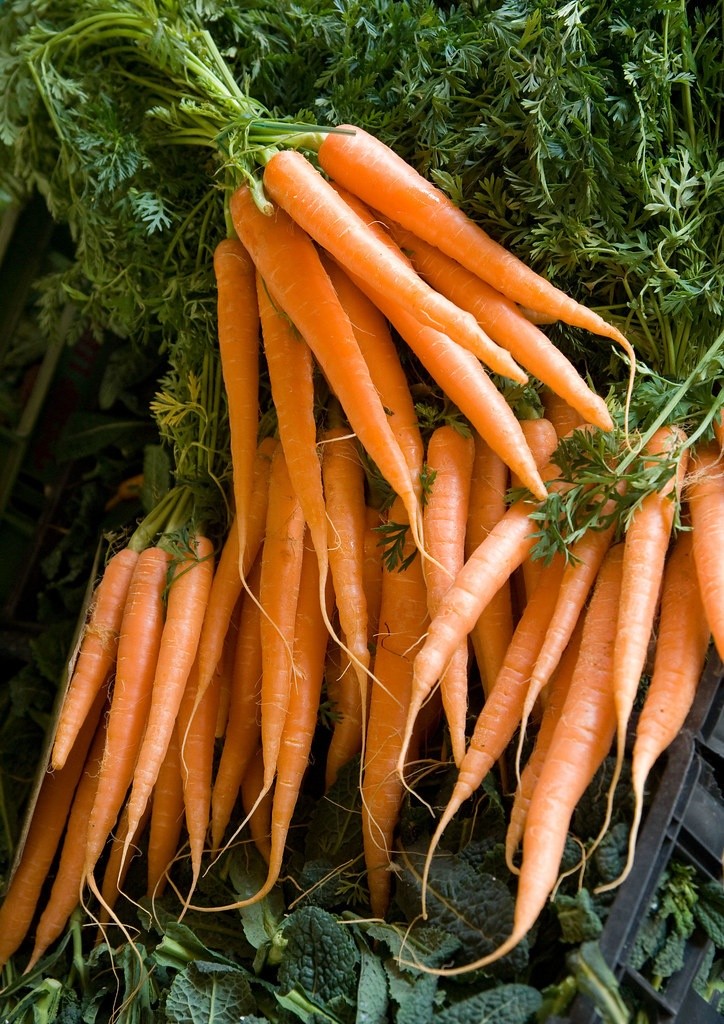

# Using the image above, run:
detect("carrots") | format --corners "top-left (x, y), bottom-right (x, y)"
top-left (0, 125), bottom-right (723, 971)
